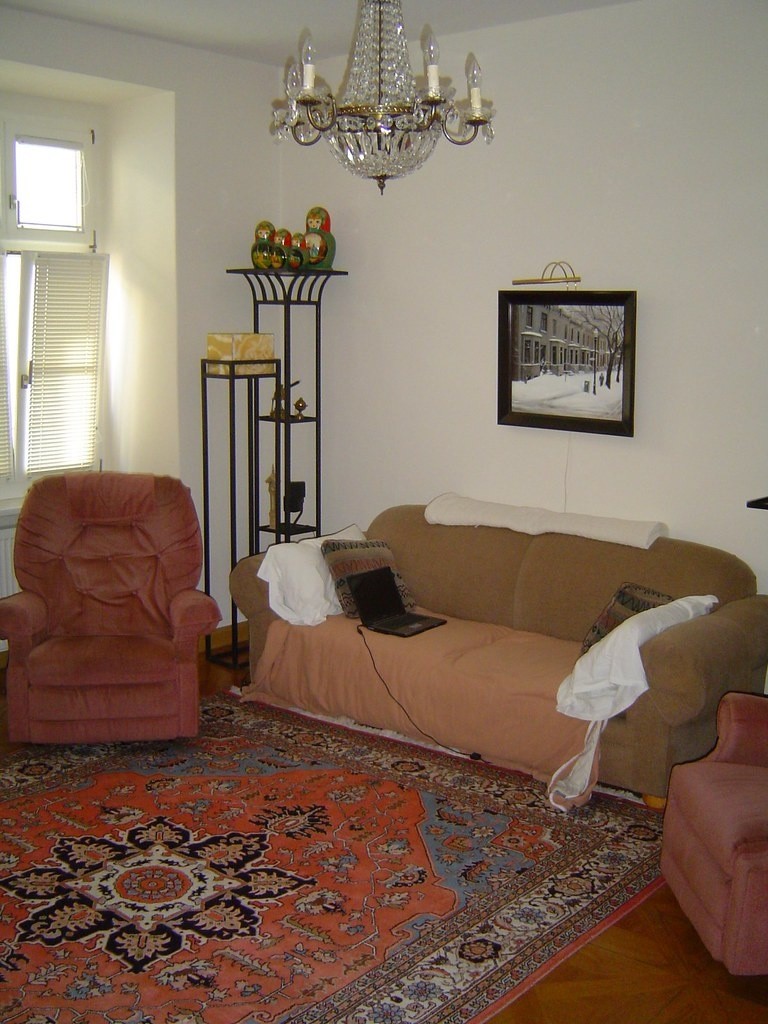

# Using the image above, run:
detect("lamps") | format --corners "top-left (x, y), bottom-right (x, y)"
top-left (267, 0), bottom-right (495, 197)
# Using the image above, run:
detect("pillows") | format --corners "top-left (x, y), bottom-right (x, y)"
top-left (260, 525), bottom-right (368, 627)
top-left (323, 540), bottom-right (417, 618)
top-left (581, 581), bottom-right (672, 659)
top-left (557, 594), bottom-right (720, 720)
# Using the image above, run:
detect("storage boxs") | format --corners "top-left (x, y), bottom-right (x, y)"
top-left (207, 331), bottom-right (275, 376)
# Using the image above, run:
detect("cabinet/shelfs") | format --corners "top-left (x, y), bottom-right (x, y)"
top-left (201, 265), bottom-right (348, 669)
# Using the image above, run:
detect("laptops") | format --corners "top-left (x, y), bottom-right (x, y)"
top-left (346, 566), bottom-right (448, 638)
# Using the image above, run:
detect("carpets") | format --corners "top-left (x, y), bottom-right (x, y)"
top-left (0, 690), bottom-right (665, 1024)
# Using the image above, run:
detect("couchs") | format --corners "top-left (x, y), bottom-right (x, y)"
top-left (230, 504), bottom-right (768, 809)
top-left (658, 693), bottom-right (768, 976)
top-left (0, 471), bottom-right (223, 744)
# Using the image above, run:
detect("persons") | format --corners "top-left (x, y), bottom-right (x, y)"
top-left (599, 373), bottom-right (604, 387)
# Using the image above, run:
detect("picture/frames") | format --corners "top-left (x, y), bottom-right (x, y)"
top-left (497, 289), bottom-right (635, 439)
